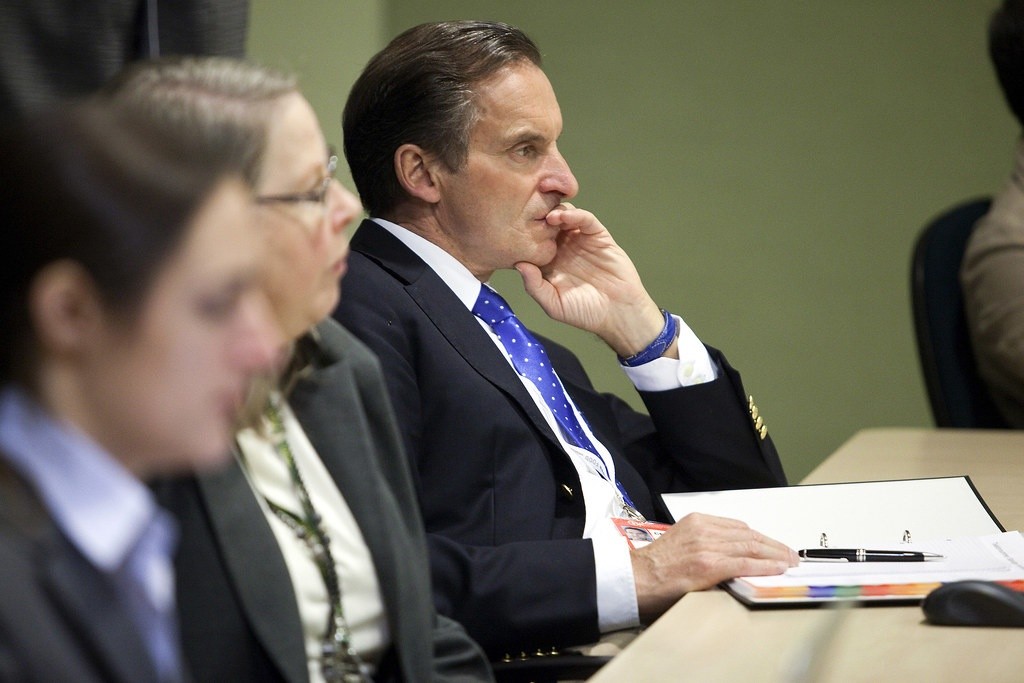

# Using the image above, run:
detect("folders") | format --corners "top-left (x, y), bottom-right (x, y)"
top-left (656, 471), bottom-right (1024, 609)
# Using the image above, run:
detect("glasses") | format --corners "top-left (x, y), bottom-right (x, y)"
top-left (248, 140), bottom-right (340, 212)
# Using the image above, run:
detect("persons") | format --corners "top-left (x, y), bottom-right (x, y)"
top-left (0, 94), bottom-right (280, 683)
top-left (129, 58), bottom-right (493, 683)
top-left (625, 528), bottom-right (651, 549)
top-left (327, 19), bottom-right (800, 683)
top-left (960, 0), bottom-right (1024, 393)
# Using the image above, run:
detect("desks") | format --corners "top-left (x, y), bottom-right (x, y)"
top-left (588, 428), bottom-right (1024, 683)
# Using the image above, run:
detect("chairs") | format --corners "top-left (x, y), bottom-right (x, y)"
top-left (909, 193), bottom-right (1024, 431)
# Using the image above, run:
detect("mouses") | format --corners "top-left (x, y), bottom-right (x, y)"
top-left (920, 579), bottom-right (1024, 628)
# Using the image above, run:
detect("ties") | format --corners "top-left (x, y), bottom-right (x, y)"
top-left (117, 511), bottom-right (184, 683)
top-left (472, 283), bottom-right (637, 511)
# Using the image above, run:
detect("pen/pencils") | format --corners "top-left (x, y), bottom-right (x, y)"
top-left (799, 547), bottom-right (944, 562)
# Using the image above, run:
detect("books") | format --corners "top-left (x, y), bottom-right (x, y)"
top-left (662, 473), bottom-right (1024, 612)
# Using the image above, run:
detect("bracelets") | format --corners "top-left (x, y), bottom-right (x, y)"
top-left (616, 308), bottom-right (678, 368)
